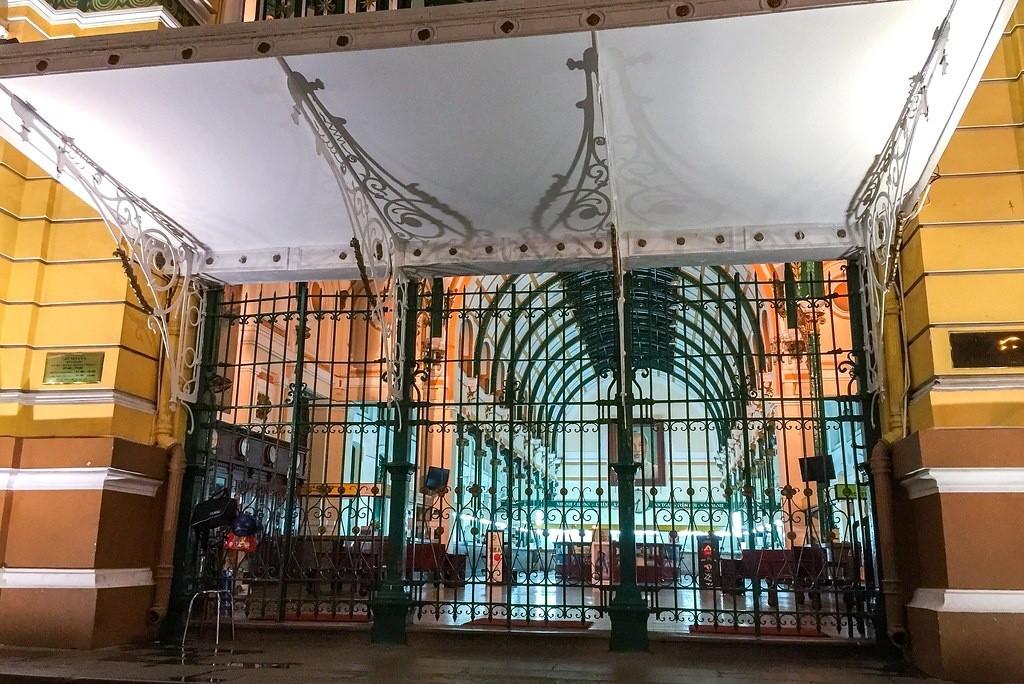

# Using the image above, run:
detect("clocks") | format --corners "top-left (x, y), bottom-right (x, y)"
top-left (633, 488), bottom-right (650, 513)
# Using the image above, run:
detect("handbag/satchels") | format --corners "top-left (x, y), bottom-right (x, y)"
top-left (223, 531), bottom-right (257, 553)
top-left (232, 506), bottom-right (265, 535)
top-left (189, 489), bottom-right (238, 530)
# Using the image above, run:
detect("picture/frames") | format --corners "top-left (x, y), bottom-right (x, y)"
top-left (606, 421), bottom-right (667, 486)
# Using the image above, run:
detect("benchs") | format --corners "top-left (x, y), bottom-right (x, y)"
top-left (742, 547), bottom-right (866, 610)
top-left (407, 543), bottom-right (446, 589)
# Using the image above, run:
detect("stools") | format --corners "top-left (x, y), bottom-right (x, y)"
top-left (181, 588), bottom-right (235, 645)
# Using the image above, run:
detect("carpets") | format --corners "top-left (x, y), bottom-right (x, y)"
top-left (462, 618), bottom-right (595, 630)
top-left (688, 625), bottom-right (831, 637)
top-left (250, 611), bottom-right (374, 623)
